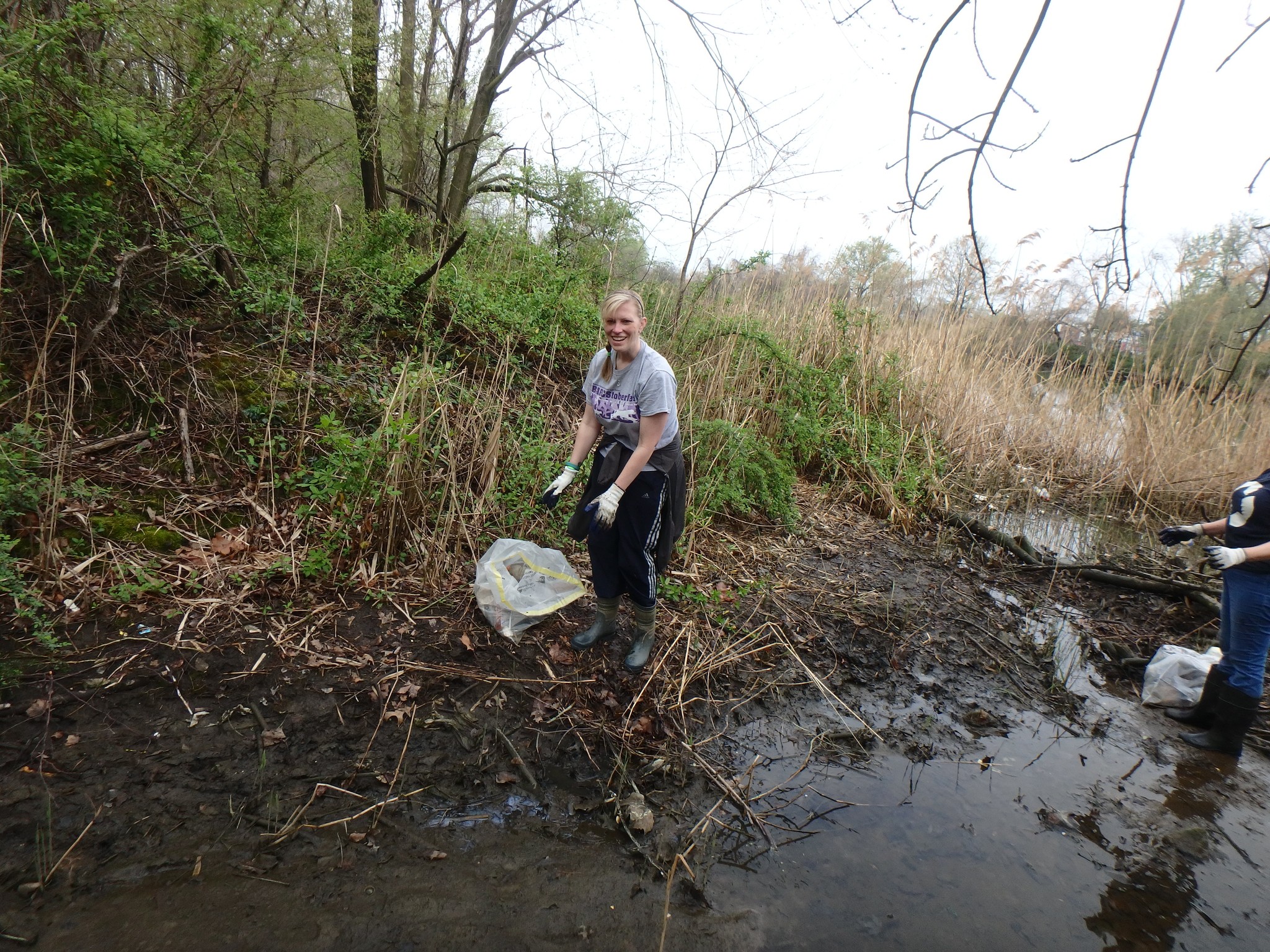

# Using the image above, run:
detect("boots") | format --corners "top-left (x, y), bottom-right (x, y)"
top-left (570, 595), bottom-right (622, 650)
top-left (625, 597), bottom-right (657, 669)
top-left (1164, 663), bottom-right (1234, 730)
top-left (1178, 679), bottom-right (1262, 755)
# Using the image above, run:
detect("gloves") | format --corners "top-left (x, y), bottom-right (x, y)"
top-left (1156, 523), bottom-right (1204, 548)
top-left (584, 483), bottom-right (626, 532)
top-left (1202, 546), bottom-right (1246, 571)
top-left (542, 465), bottom-right (579, 509)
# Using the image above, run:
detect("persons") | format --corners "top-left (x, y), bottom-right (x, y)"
top-left (540, 289), bottom-right (686, 672)
top-left (1157, 468), bottom-right (1270, 756)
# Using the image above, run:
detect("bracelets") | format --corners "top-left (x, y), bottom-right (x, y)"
top-left (565, 462), bottom-right (579, 471)
